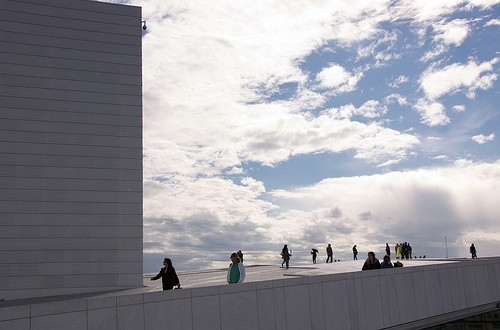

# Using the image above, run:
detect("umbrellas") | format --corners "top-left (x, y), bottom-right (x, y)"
top-left (311, 249), bottom-right (318, 253)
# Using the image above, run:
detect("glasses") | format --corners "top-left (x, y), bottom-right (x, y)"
top-left (163, 261), bottom-right (167, 263)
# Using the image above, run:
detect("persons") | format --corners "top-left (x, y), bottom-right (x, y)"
top-left (352, 245), bottom-right (358, 260)
top-left (381, 255), bottom-right (394, 269)
top-left (151, 257), bottom-right (180, 291)
top-left (385, 243), bottom-right (391, 256)
top-left (362, 252), bottom-right (381, 270)
top-left (393, 261), bottom-right (403, 268)
top-left (423, 255), bottom-right (426, 258)
top-left (419, 256), bottom-right (422, 258)
top-left (237, 250), bottom-right (243, 263)
top-left (395, 242), bottom-right (413, 260)
top-left (226, 253), bottom-right (245, 284)
top-left (415, 256), bottom-right (417, 258)
top-left (282, 244), bottom-right (292, 266)
top-left (470, 243), bottom-right (477, 259)
top-left (326, 244), bottom-right (333, 263)
top-left (311, 250), bottom-right (317, 264)
